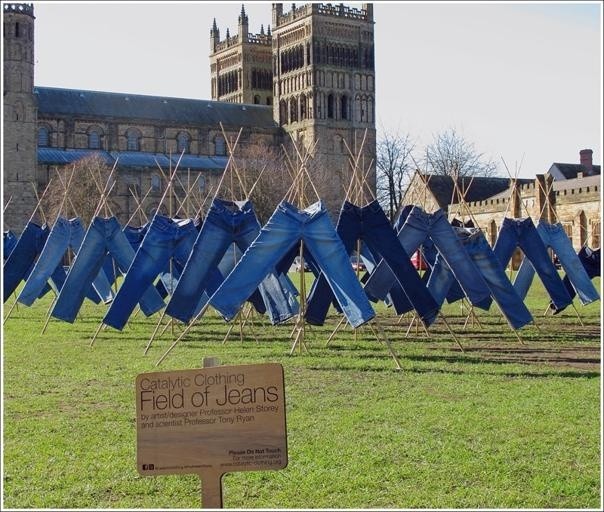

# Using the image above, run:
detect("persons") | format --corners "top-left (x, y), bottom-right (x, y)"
top-left (553, 253), bottom-right (559, 270)
